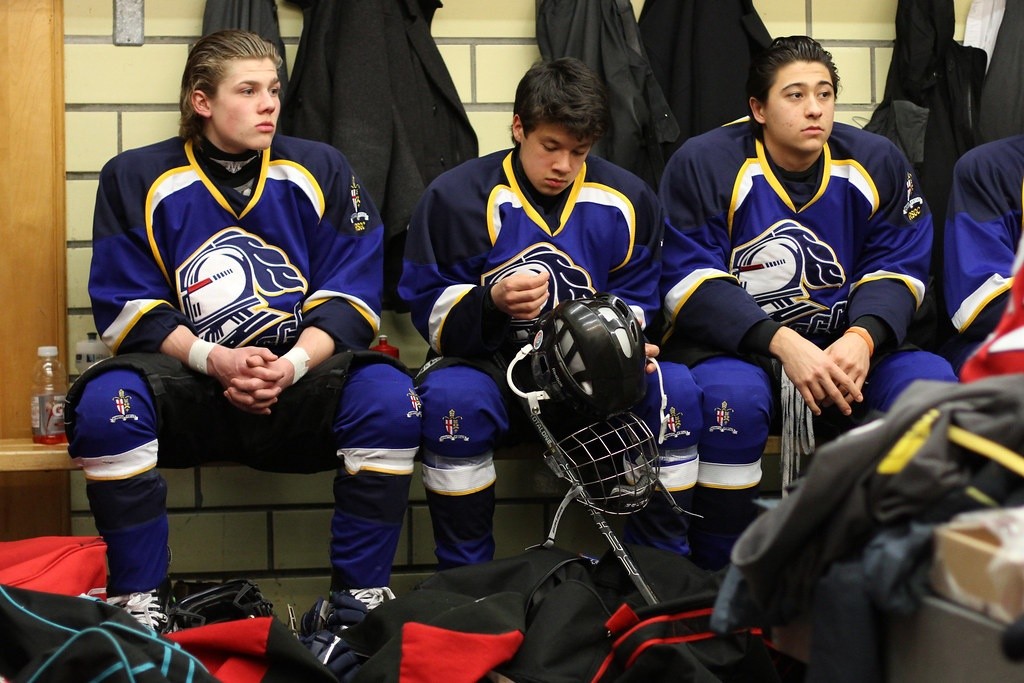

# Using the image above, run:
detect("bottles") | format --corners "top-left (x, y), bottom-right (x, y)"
top-left (75, 332), bottom-right (110, 374)
top-left (369, 335), bottom-right (400, 361)
top-left (29, 346), bottom-right (68, 444)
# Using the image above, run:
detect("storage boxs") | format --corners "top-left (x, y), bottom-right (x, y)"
top-left (933, 503), bottom-right (1024, 625)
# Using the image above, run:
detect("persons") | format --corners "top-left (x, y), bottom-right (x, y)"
top-left (657, 35), bottom-right (960, 571)
top-left (64, 29), bottom-right (423, 638)
top-left (941, 133), bottom-right (1024, 389)
top-left (398, 56), bottom-right (704, 574)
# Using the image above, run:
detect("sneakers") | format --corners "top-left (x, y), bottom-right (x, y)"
top-left (328, 586), bottom-right (396, 614)
top-left (120, 574), bottom-right (171, 634)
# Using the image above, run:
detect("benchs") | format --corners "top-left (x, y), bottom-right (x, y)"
top-left (0, 425), bottom-right (783, 471)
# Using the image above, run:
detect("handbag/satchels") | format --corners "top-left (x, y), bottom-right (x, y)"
top-left (0, 536), bottom-right (815, 683)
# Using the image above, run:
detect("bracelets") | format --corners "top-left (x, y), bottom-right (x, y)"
top-left (189, 339), bottom-right (214, 374)
top-left (281, 347), bottom-right (311, 385)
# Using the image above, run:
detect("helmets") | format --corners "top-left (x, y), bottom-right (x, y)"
top-left (529, 293), bottom-right (659, 514)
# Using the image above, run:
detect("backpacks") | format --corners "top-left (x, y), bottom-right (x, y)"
top-left (733, 373), bottom-right (1024, 619)
